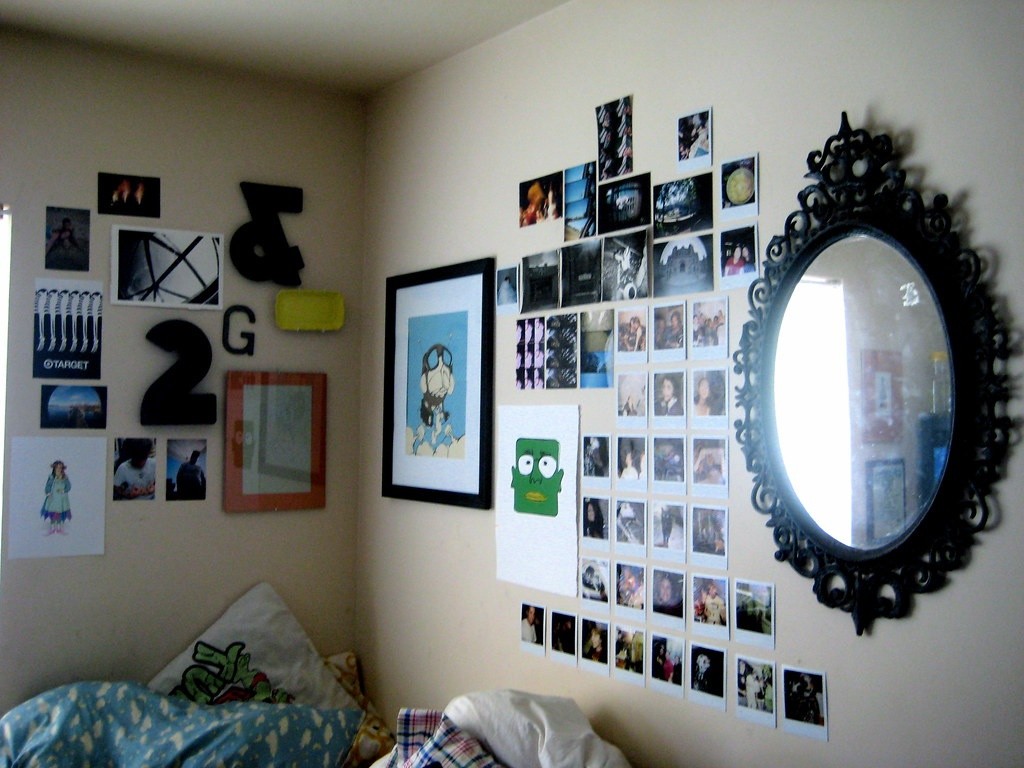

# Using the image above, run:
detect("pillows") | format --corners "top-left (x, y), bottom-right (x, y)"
top-left (322, 650), bottom-right (397, 768)
top-left (148, 582), bottom-right (360, 708)
top-left (0, 679), bottom-right (366, 768)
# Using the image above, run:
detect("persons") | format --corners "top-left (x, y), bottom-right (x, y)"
top-left (51, 217), bottom-right (78, 248)
top-left (504, 101), bottom-right (825, 727)
top-left (176, 449), bottom-right (206, 490)
top-left (114, 439), bottom-right (156, 499)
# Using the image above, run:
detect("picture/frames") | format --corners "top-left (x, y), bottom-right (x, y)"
top-left (381, 257), bottom-right (496, 511)
top-left (222, 369), bottom-right (329, 514)
top-left (110, 223), bottom-right (225, 310)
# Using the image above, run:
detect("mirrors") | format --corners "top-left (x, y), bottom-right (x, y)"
top-left (732, 112), bottom-right (1006, 637)
